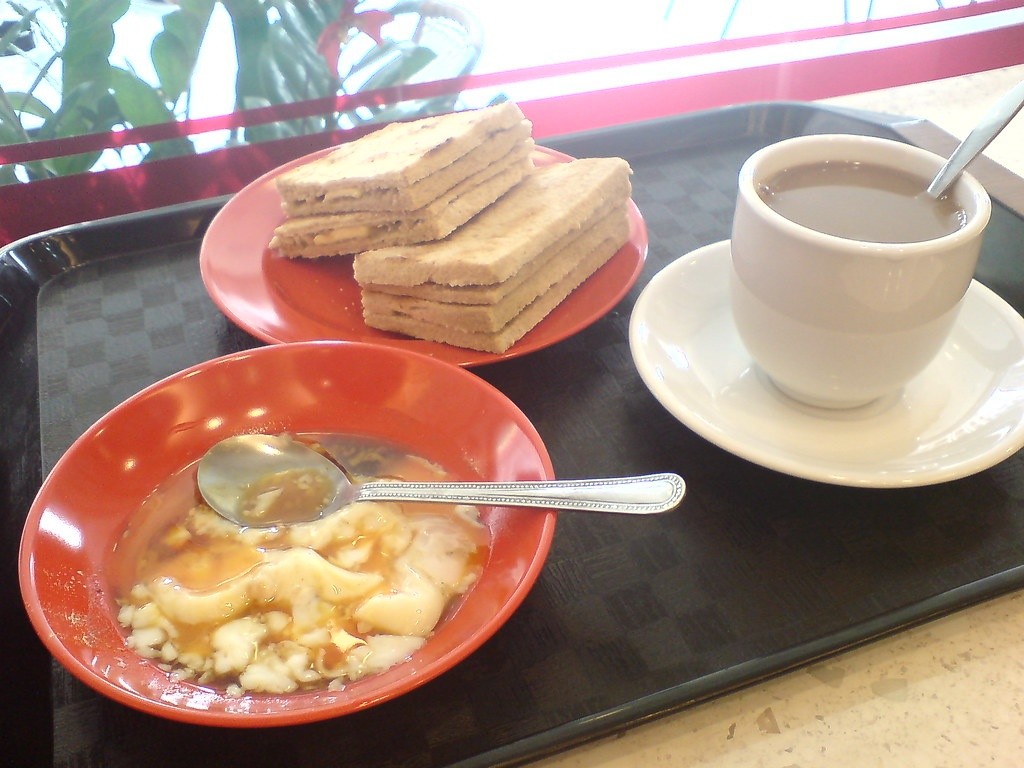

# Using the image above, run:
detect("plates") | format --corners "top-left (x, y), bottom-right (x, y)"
top-left (629, 239), bottom-right (1024, 487)
top-left (199, 139), bottom-right (649, 367)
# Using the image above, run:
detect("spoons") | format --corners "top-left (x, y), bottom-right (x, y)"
top-left (198, 432), bottom-right (687, 528)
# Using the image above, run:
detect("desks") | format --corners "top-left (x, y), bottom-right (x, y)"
top-left (523, 61), bottom-right (1024, 768)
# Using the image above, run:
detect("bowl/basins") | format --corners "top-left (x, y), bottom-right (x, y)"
top-left (19, 341), bottom-right (555, 726)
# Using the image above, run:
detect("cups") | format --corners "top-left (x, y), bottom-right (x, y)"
top-left (730, 133), bottom-right (993, 409)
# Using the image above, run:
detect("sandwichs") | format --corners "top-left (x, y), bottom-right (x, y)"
top-left (267, 97), bottom-right (633, 352)
